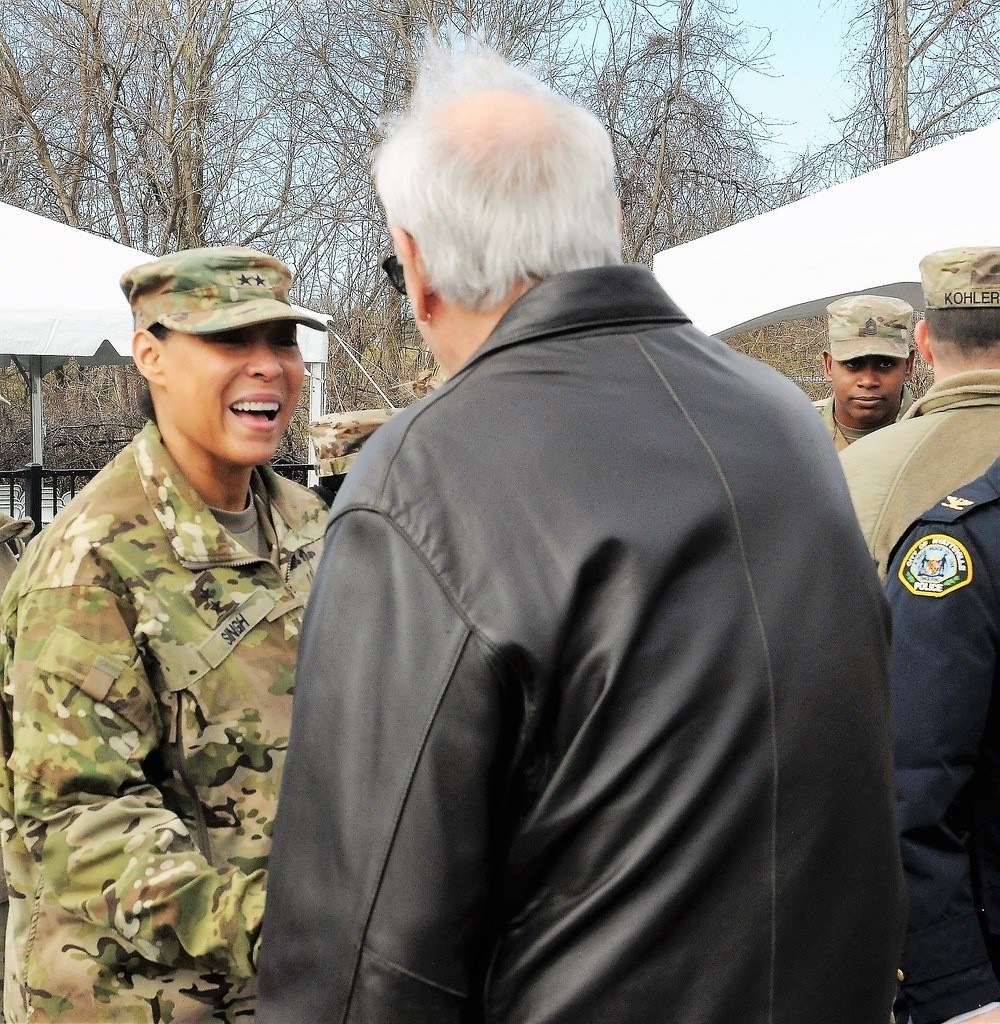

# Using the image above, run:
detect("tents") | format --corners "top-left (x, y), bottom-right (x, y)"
top-left (2, 196), bottom-right (330, 495)
top-left (648, 110), bottom-right (1000, 341)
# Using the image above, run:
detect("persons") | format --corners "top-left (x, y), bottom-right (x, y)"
top-left (257, 26), bottom-right (907, 1024)
top-left (812, 295), bottom-right (917, 452)
top-left (1, 245), bottom-right (334, 1023)
top-left (835, 244), bottom-right (1000, 582)
top-left (880, 447), bottom-right (1000, 1024)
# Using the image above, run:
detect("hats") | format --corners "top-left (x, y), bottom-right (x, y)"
top-left (919, 246), bottom-right (1000, 309)
top-left (120, 244), bottom-right (327, 335)
top-left (825, 295), bottom-right (914, 362)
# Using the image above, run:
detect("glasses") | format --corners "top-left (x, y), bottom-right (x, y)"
top-left (382, 255), bottom-right (406, 294)
